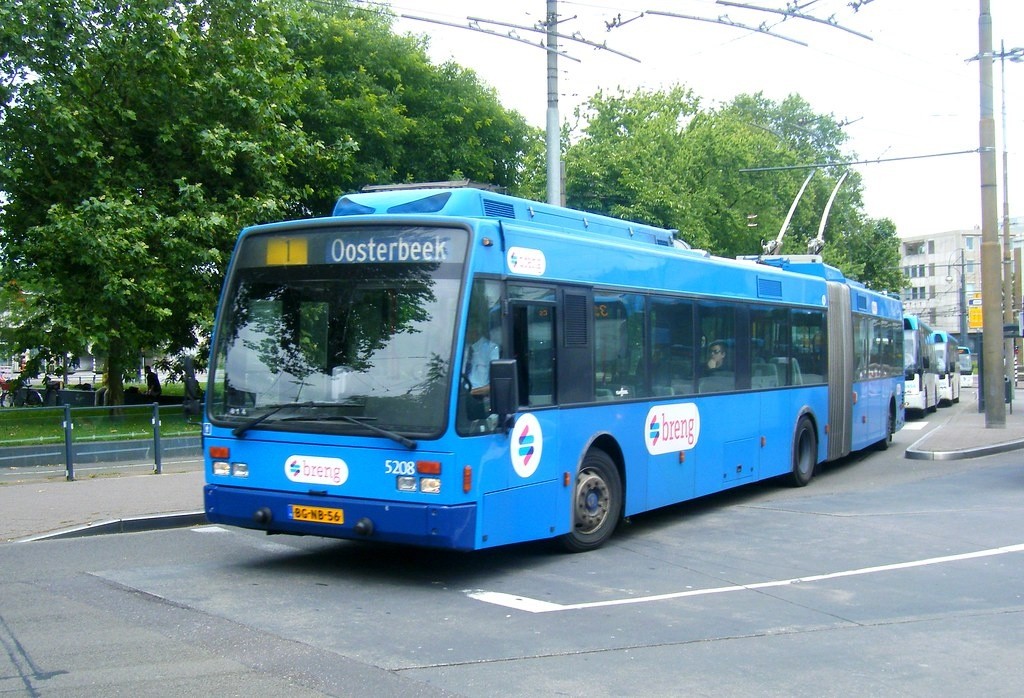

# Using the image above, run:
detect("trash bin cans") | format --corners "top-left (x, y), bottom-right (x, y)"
top-left (1005, 376), bottom-right (1011, 403)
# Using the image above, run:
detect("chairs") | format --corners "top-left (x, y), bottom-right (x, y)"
top-left (596, 355), bottom-right (803, 400)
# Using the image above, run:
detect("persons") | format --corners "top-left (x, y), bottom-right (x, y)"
top-left (144, 365), bottom-right (162, 402)
top-left (95, 366), bottom-right (110, 406)
top-left (699, 343), bottom-right (728, 378)
top-left (455, 313), bottom-right (500, 414)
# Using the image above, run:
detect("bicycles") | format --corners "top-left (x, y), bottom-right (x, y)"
top-left (0, 379), bottom-right (44, 409)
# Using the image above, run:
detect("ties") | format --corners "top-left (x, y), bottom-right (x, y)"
top-left (462, 346), bottom-right (473, 386)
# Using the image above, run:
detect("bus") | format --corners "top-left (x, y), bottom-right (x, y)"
top-left (934, 330), bottom-right (961, 407)
top-left (958, 347), bottom-right (973, 376)
top-left (185, 167), bottom-right (905, 553)
top-left (903, 315), bottom-right (941, 418)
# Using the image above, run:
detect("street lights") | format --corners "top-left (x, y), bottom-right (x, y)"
top-left (945, 248), bottom-right (970, 348)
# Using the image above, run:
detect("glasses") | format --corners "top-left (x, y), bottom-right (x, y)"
top-left (710, 350), bottom-right (722, 354)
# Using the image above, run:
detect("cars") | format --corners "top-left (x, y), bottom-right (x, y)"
top-left (45, 373), bottom-right (64, 390)
